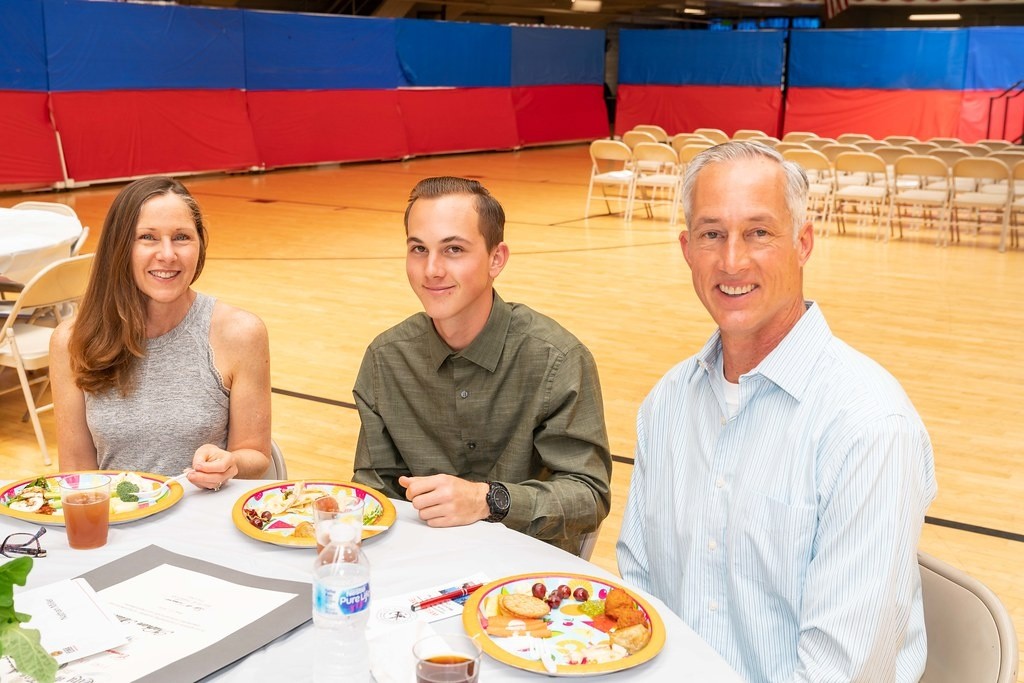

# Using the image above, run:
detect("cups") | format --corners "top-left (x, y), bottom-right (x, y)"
top-left (313, 495), bottom-right (364, 564)
top-left (58, 474), bottom-right (111, 550)
top-left (412, 633), bottom-right (482, 683)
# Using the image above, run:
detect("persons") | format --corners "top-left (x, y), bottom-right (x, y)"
top-left (352, 175), bottom-right (614, 558)
top-left (615, 137), bottom-right (937, 683)
top-left (49, 174), bottom-right (273, 491)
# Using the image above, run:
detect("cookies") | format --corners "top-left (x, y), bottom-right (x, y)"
top-left (498, 595), bottom-right (549, 619)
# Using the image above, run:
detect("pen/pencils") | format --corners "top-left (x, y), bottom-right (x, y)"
top-left (411, 583), bottom-right (484, 615)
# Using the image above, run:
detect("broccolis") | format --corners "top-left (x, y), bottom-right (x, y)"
top-left (111, 481), bottom-right (139, 501)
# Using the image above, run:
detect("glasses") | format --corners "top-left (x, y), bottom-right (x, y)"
top-left (0, 527), bottom-right (46, 558)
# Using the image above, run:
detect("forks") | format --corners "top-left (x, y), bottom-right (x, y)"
top-left (128, 459), bottom-right (221, 498)
top-left (531, 638), bottom-right (557, 674)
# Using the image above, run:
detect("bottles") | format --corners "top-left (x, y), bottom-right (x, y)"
top-left (309, 530), bottom-right (370, 683)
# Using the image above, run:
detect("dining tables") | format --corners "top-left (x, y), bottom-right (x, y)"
top-left (0, 472), bottom-right (743, 683)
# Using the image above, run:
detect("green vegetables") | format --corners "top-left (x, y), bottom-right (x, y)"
top-left (0, 557), bottom-right (57, 683)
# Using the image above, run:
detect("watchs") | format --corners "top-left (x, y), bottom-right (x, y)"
top-left (480, 479), bottom-right (511, 523)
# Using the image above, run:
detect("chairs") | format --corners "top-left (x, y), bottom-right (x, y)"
top-left (919, 551), bottom-right (1019, 683)
top-left (26, 226), bottom-right (90, 326)
top-left (0, 254), bottom-right (98, 466)
top-left (0, 201), bottom-right (79, 300)
top-left (582, 125), bottom-right (1024, 253)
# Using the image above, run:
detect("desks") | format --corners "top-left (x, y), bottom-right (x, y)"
top-left (0, 206), bottom-right (82, 282)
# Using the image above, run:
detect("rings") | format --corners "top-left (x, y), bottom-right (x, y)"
top-left (213, 482), bottom-right (222, 492)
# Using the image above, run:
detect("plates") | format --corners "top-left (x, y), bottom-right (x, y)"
top-left (234, 479), bottom-right (396, 547)
top-left (0, 470), bottom-right (184, 526)
top-left (462, 572), bottom-right (666, 676)
